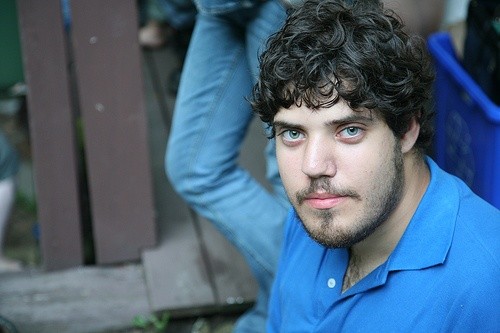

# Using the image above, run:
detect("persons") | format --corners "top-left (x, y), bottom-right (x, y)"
top-left (250, 0), bottom-right (500, 333)
top-left (161, 0), bottom-right (297, 333)
top-left (0, 133), bottom-right (25, 274)
top-left (136, 0), bottom-right (199, 135)
